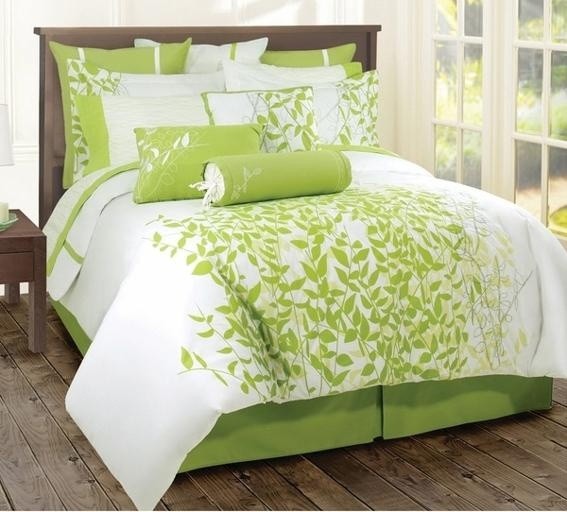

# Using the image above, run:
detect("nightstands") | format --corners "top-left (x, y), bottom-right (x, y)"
top-left (0, 209), bottom-right (47, 353)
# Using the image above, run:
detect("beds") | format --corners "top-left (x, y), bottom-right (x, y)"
top-left (31, 24), bottom-right (567, 476)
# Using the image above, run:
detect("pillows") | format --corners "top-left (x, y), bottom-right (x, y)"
top-left (49, 34), bottom-right (383, 188)
top-left (188, 150), bottom-right (353, 205)
top-left (132, 123), bottom-right (264, 203)
